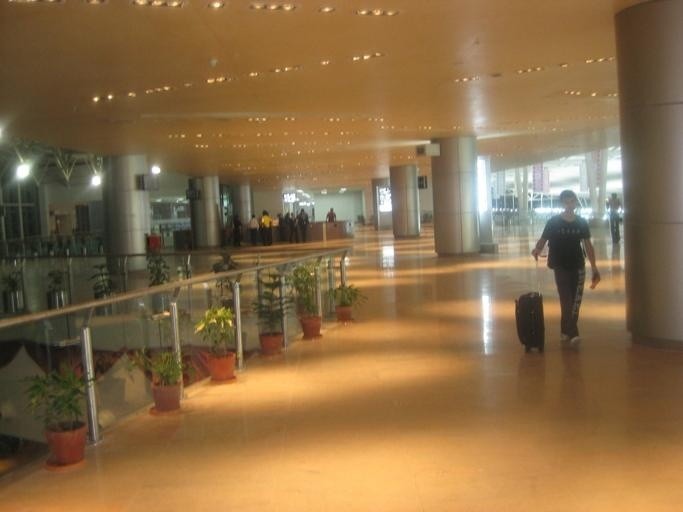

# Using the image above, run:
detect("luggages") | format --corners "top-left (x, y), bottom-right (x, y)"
top-left (513, 254), bottom-right (547, 352)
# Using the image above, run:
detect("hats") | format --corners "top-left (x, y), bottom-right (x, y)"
top-left (561, 189), bottom-right (583, 209)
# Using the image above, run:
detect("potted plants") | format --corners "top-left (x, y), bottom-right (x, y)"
top-left (133, 340), bottom-right (188, 413)
top-left (295, 260), bottom-right (322, 337)
top-left (323, 285), bottom-right (364, 323)
top-left (18, 361), bottom-right (97, 463)
top-left (252, 272), bottom-right (297, 352)
top-left (193, 302), bottom-right (236, 379)
top-left (9, 253), bottom-right (243, 315)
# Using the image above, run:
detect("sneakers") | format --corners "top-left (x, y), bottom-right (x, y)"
top-left (559, 332), bottom-right (568, 341)
top-left (570, 336), bottom-right (581, 348)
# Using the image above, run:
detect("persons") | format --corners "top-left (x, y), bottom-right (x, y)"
top-left (606, 192), bottom-right (623, 245)
top-left (528, 187), bottom-right (603, 351)
top-left (231, 206), bottom-right (337, 248)
